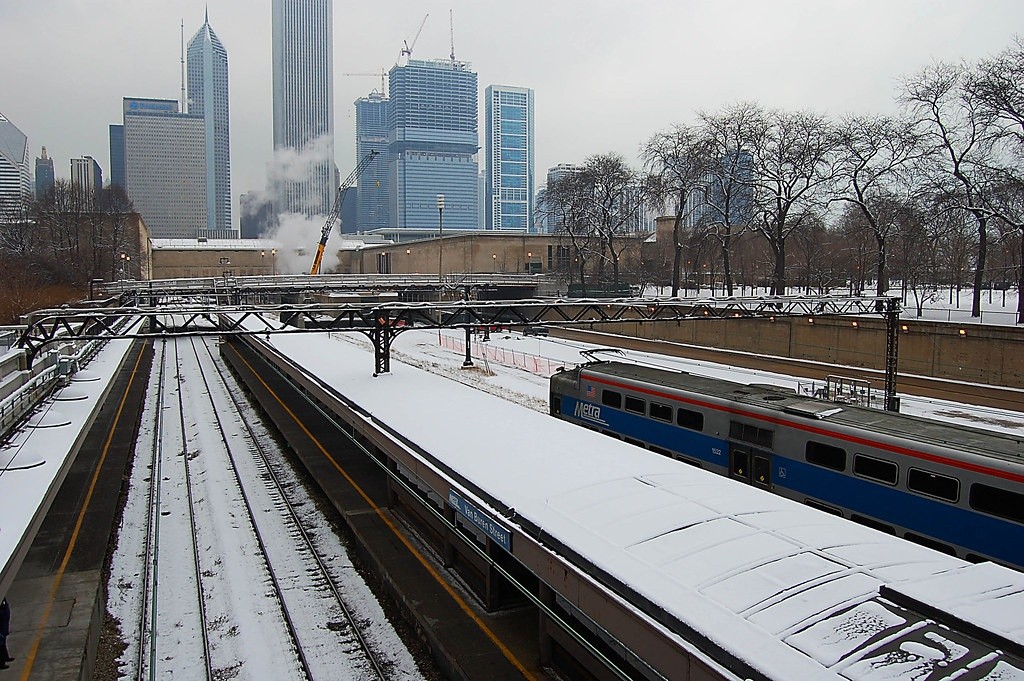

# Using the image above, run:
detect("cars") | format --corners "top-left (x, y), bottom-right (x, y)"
top-left (471, 319), bottom-right (507, 333)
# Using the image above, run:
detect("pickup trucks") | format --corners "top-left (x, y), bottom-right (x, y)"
top-left (522, 324), bottom-right (550, 337)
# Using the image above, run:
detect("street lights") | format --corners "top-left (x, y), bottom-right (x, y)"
top-left (436, 194), bottom-right (444, 327)
top-left (528, 252), bottom-right (531, 274)
top-left (272, 249), bottom-right (275, 283)
top-left (407, 248), bottom-right (410, 274)
top-left (492, 253), bottom-right (496, 274)
top-left (119, 251), bottom-right (130, 292)
top-left (219, 258), bottom-right (230, 288)
top-left (261, 250), bottom-right (264, 280)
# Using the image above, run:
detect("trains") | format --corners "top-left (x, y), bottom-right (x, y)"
top-left (549, 348), bottom-right (1024, 574)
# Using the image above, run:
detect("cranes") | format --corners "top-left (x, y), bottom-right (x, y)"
top-left (450, 9), bottom-right (455, 59)
top-left (310, 149), bottom-right (381, 275)
top-left (343, 67), bottom-right (389, 96)
top-left (397, 13), bottom-right (430, 64)
